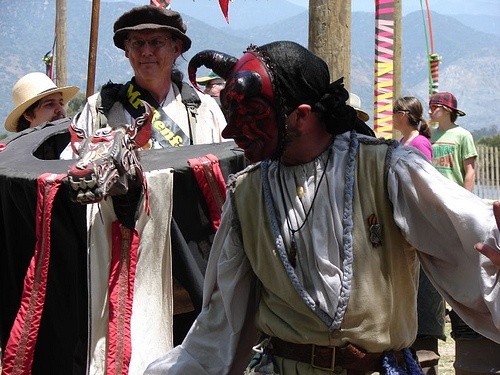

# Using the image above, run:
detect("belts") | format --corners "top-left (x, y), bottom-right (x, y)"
top-left (258, 334), bottom-right (419, 370)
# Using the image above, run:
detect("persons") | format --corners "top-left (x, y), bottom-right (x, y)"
top-left (346, 92), bottom-right (478, 191)
top-left (59, 6), bottom-right (236, 160)
top-left (4, 72), bottom-right (81, 133)
top-left (143, 40), bottom-right (500, 375)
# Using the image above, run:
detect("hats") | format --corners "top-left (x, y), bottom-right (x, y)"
top-left (4, 71), bottom-right (81, 132)
top-left (115, 4), bottom-right (192, 56)
top-left (196, 68), bottom-right (226, 86)
top-left (430, 91), bottom-right (467, 117)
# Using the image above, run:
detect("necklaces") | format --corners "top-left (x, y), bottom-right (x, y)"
top-left (278, 148), bottom-right (333, 269)
top-left (150, 92), bottom-right (168, 150)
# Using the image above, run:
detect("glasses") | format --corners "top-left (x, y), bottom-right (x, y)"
top-left (127, 35), bottom-right (177, 50)
top-left (393, 107), bottom-right (411, 119)
